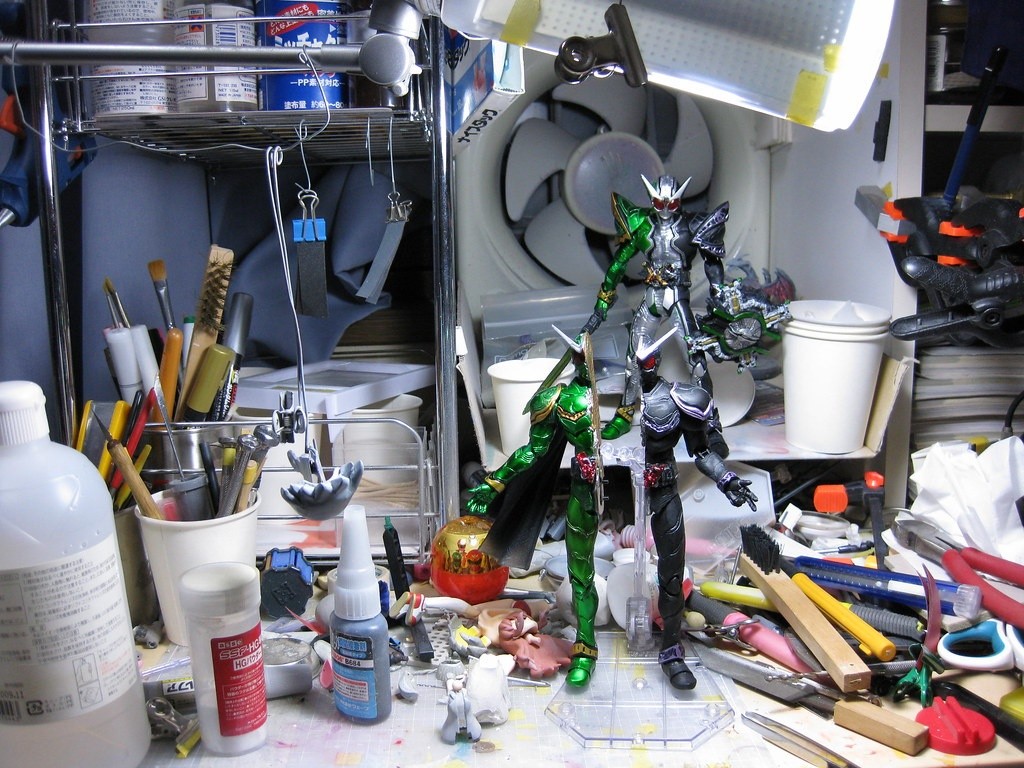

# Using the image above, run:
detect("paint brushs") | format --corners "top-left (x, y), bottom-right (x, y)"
top-left (97, 259), bottom-right (185, 409)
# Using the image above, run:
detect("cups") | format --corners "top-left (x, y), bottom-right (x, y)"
top-left (136, 484), bottom-right (257, 643)
top-left (657, 307), bottom-right (755, 426)
top-left (332, 395), bottom-right (424, 481)
top-left (125, 420), bottom-right (240, 487)
top-left (783, 299), bottom-right (889, 453)
top-left (488, 355), bottom-right (577, 450)
top-left (233, 405), bottom-right (321, 515)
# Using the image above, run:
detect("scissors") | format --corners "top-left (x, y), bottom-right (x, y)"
top-left (937, 616), bottom-right (1024, 674)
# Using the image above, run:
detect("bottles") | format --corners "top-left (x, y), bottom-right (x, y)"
top-left (179, 562), bottom-right (267, 755)
top-left (0, 381), bottom-right (150, 768)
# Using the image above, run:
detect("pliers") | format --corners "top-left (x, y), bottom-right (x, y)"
top-left (890, 519), bottom-right (1024, 630)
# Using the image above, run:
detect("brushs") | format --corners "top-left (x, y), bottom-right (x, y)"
top-left (178, 245), bottom-right (237, 423)
top-left (738, 522), bottom-right (873, 700)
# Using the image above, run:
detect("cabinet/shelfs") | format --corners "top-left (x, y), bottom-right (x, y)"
top-left (772, 1), bottom-right (1024, 550)
top-left (22, 0), bottom-right (458, 567)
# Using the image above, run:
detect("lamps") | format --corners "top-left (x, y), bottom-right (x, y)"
top-left (0, 0), bottom-right (895, 135)
